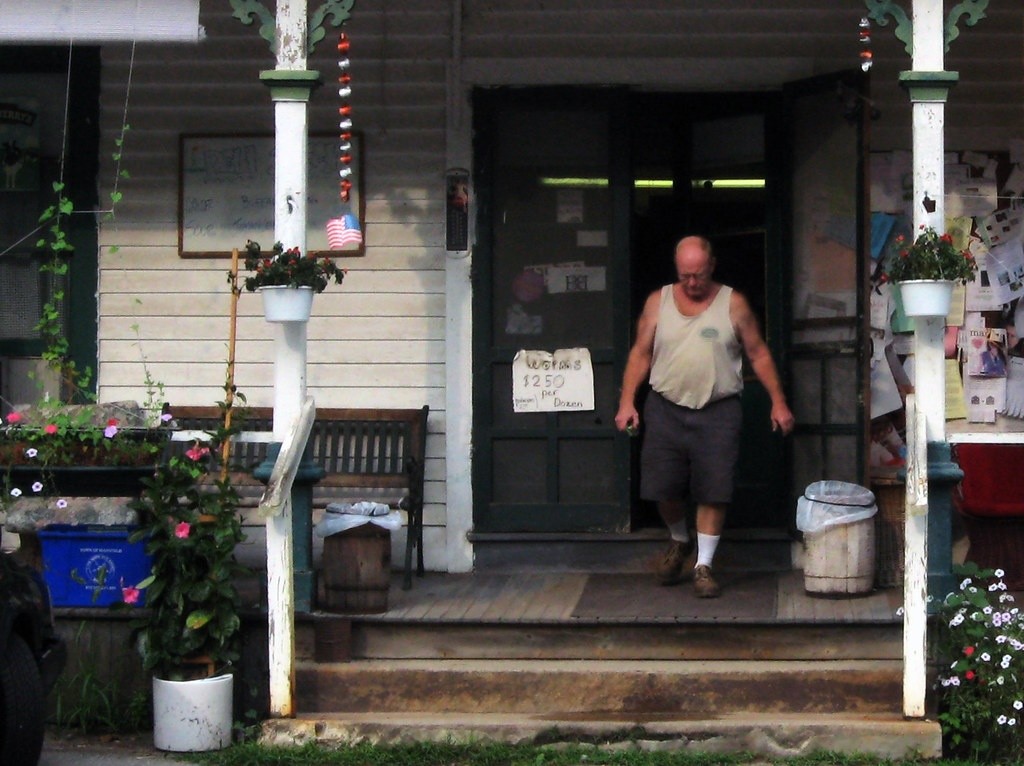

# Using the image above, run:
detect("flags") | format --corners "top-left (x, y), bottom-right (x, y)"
top-left (326, 213), bottom-right (362, 250)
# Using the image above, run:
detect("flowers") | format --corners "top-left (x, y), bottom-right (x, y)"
top-left (222, 237), bottom-right (351, 300)
top-left (867, 223), bottom-right (980, 296)
top-left (893, 556), bottom-right (1024, 766)
top-left (125, 338), bottom-right (253, 686)
top-left (0, 120), bottom-right (178, 518)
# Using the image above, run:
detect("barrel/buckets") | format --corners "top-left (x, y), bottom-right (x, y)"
top-left (322, 503), bottom-right (392, 616)
top-left (804, 486), bottom-right (876, 599)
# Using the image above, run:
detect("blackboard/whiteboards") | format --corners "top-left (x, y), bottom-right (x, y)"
top-left (177, 128), bottom-right (368, 257)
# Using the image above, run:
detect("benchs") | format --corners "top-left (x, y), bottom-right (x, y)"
top-left (150, 399), bottom-right (435, 593)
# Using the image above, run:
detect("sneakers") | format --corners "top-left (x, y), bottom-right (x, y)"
top-left (693, 564), bottom-right (720, 597)
top-left (655, 530), bottom-right (694, 585)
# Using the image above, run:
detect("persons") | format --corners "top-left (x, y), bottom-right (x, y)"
top-left (614, 236), bottom-right (795, 599)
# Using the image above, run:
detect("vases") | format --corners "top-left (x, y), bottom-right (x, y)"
top-left (895, 276), bottom-right (956, 319)
top-left (148, 672), bottom-right (236, 755)
top-left (0, 428), bottom-right (173, 498)
top-left (256, 284), bottom-right (314, 325)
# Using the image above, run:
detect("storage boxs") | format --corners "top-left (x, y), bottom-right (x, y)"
top-left (33, 520), bottom-right (155, 611)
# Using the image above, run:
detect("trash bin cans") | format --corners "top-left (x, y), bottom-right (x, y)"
top-left (317, 502), bottom-right (395, 610)
top-left (800, 480), bottom-right (878, 596)
